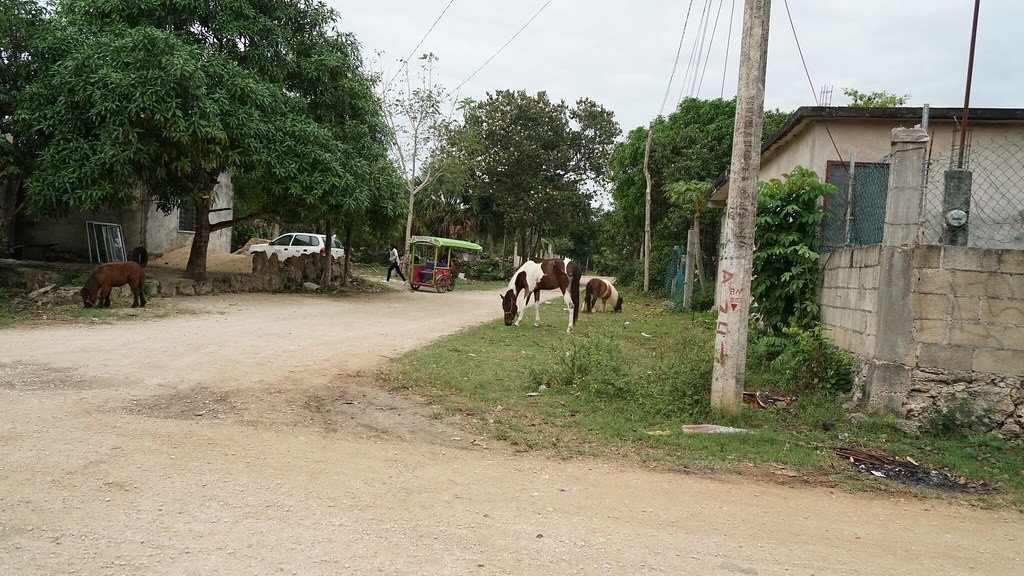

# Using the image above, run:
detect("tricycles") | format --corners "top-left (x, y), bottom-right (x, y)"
top-left (409, 235), bottom-right (458, 294)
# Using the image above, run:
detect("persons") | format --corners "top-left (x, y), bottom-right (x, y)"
top-left (382, 243), bottom-right (407, 285)
top-left (440, 248), bottom-right (455, 267)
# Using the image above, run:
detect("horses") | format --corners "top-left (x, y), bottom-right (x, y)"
top-left (80, 261), bottom-right (148, 308)
top-left (500, 257), bottom-right (623, 331)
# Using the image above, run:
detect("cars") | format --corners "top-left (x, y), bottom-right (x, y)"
top-left (249, 234), bottom-right (345, 261)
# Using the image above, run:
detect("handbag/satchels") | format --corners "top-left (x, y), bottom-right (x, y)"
top-left (390, 262), bottom-right (397, 268)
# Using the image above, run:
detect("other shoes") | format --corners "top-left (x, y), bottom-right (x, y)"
top-left (402, 280), bottom-right (408, 285)
top-left (382, 280), bottom-right (389, 283)
